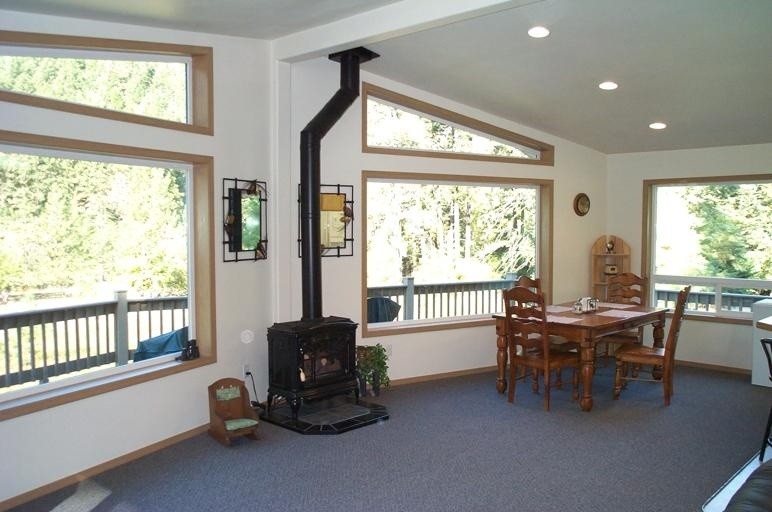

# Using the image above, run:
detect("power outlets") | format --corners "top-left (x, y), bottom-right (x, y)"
top-left (242, 365), bottom-right (251, 379)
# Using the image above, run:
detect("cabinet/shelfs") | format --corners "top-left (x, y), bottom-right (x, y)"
top-left (503, 274), bottom-right (692, 412)
top-left (590, 234), bottom-right (631, 303)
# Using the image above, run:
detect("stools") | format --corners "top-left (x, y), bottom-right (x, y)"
top-left (207, 376), bottom-right (260, 449)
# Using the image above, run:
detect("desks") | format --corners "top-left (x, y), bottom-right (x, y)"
top-left (749, 298), bottom-right (771, 387)
top-left (491, 301), bottom-right (671, 411)
